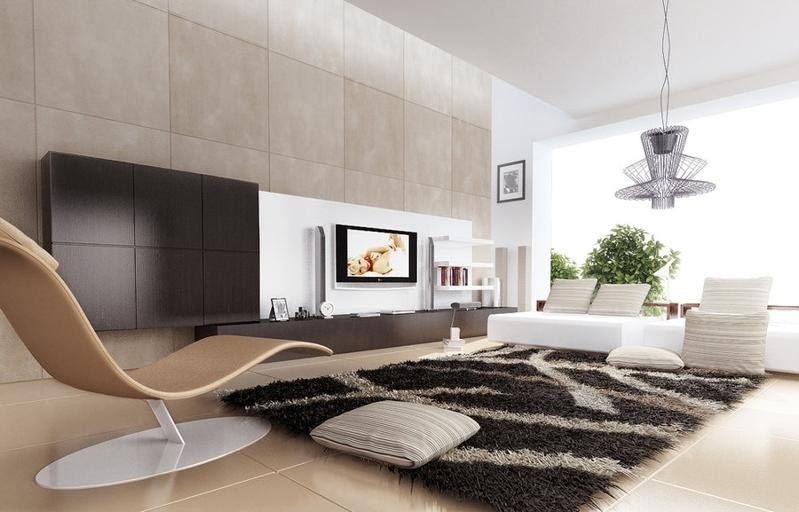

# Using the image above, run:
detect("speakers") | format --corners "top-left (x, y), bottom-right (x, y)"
top-left (315, 226), bottom-right (326, 318)
top-left (425, 237), bottom-right (435, 310)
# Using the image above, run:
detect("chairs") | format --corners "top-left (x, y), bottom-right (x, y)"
top-left (1, 215), bottom-right (334, 491)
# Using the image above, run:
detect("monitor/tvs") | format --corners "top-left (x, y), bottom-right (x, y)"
top-left (332, 222), bottom-right (419, 291)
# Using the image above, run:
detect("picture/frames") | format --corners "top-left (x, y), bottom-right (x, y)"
top-left (269, 297), bottom-right (290, 322)
top-left (497, 159), bottom-right (525, 203)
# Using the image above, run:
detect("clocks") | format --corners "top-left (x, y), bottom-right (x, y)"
top-left (319, 300), bottom-right (334, 319)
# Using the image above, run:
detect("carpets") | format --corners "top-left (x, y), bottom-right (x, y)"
top-left (214, 343), bottom-right (771, 512)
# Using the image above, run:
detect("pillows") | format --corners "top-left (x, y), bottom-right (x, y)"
top-left (681, 310), bottom-right (770, 374)
top-left (589, 283), bottom-right (651, 318)
top-left (310, 399), bottom-right (481, 469)
top-left (605, 345), bottom-right (685, 375)
top-left (699, 278), bottom-right (770, 315)
top-left (546, 277), bottom-right (597, 314)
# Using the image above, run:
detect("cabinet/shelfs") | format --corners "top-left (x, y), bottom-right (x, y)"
top-left (429, 235), bottom-right (501, 310)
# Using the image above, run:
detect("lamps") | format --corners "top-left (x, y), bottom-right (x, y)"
top-left (614, 0), bottom-right (716, 209)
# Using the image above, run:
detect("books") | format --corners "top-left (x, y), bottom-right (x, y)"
top-left (358, 312), bottom-right (380, 317)
top-left (437, 265), bottom-right (468, 287)
top-left (381, 309), bottom-right (415, 314)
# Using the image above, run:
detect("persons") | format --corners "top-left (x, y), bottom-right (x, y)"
top-left (349, 234), bottom-right (405, 274)
